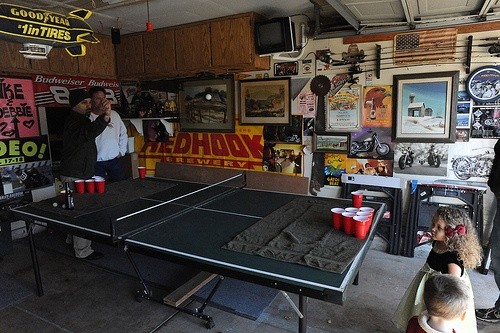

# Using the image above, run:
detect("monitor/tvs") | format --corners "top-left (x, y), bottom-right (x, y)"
top-left (254, 16), bottom-right (296, 55)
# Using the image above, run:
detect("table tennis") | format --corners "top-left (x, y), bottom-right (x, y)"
top-left (53, 203), bottom-right (57, 206)
top-left (206, 94), bottom-right (212, 100)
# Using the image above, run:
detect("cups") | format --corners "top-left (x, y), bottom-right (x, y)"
top-left (74, 175), bottom-right (106, 195)
top-left (138, 167), bottom-right (147, 180)
top-left (330, 207), bottom-right (374, 239)
top-left (351, 192), bottom-right (363, 208)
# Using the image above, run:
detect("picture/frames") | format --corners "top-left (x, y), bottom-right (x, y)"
top-left (391, 70), bottom-right (460, 143)
top-left (239, 77), bottom-right (292, 126)
top-left (176, 74), bottom-right (236, 133)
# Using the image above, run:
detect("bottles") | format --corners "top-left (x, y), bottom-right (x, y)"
top-left (65, 183), bottom-right (74, 209)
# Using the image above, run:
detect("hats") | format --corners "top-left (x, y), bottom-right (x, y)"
top-left (69, 89), bottom-right (92, 108)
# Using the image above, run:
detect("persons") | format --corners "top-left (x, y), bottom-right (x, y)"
top-left (406, 273), bottom-right (469, 333)
top-left (475, 138), bottom-right (500, 323)
top-left (87, 87), bottom-right (128, 183)
top-left (392, 207), bottom-right (482, 333)
top-left (63, 89), bottom-right (112, 259)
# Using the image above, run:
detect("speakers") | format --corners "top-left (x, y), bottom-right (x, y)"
top-left (111, 28), bottom-right (120, 44)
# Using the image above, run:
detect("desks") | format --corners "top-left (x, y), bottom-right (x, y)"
top-left (9, 175), bottom-right (388, 333)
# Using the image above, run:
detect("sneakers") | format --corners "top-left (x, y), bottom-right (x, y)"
top-left (475, 307), bottom-right (500, 322)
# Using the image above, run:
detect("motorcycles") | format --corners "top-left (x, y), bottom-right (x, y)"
top-left (418, 157), bottom-right (425, 164)
top-left (352, 127), bottom-right (390, 158)
top-left (427, 151), bottom-right (440, 167)
top-left (452, 147), bottom-right (496, 180)
top-left (398, 147), bottom-right (414, 169)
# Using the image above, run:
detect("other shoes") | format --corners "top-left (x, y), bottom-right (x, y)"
top-left (84, 251), bottom-right (103, 260)
